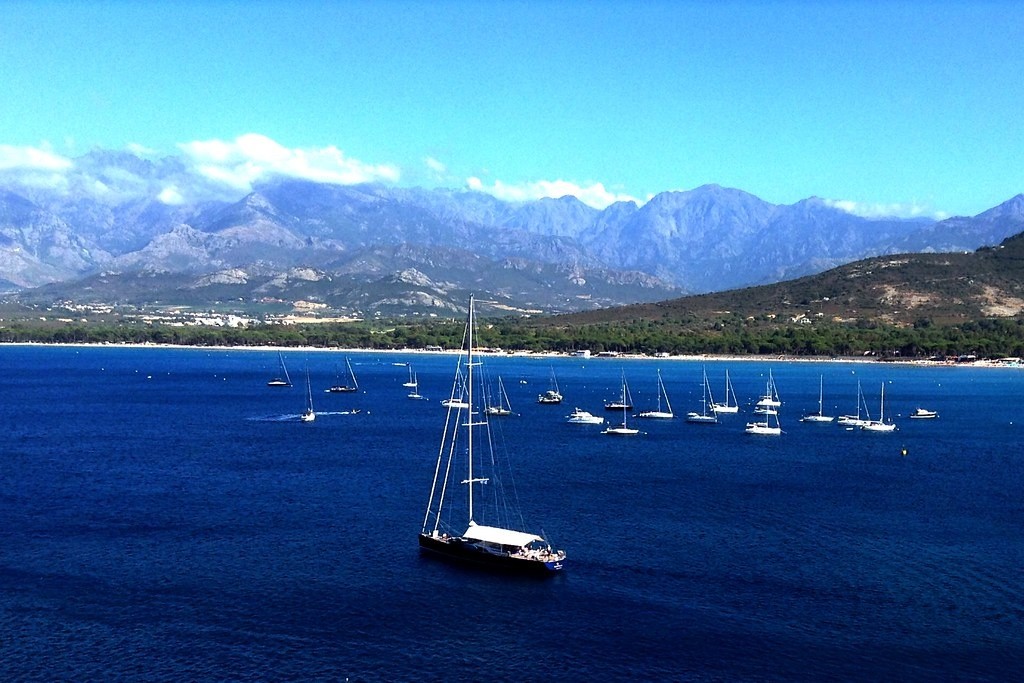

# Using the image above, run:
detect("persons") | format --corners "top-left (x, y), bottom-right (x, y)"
top-left (440, 533), bottom-right (447, 542)
top-left (519, 542), bottom-right (552, 561)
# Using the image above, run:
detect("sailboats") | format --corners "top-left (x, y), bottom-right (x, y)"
top-left (803, 374), bottom-right (835, 422)
top-left (485, 376), bottom-right (512, 415)
top-left (713, 368), bottom-right (739, 413)
top-left (440, 366), bottom-right (470, 409)
top-left (402, 364), bottom-right (417, 387)
top-left (861, 382), bottom-right (896, 432)
top-left (267, 350), bottom-right (293, 386)
top-left (639, 368), bottom-right (674, 417)
top-left (757, 368), bottom-right (781, 406)
top-left (537, 365), bottom-right (563, 404)
top-left (838, 379), bottom-right (872, 426)
top-left (419, 293), bottom-right (566, 577)
top-left (330, 356), bottom-right (359, 392)
top-left (687, 364), bottom-right (718, 422)
top-left (753, 380), bottom-right (777, 415)
top-left (605, 365), bottom-right (634, 410)
top-left (301, 359), bottom-right (315, 421)
top-left (601, 384), bottom-right (639, 434)
top-left (746, 387), bottom-right (781, 435)
top-left (408, 372), bottom-right (422, 397)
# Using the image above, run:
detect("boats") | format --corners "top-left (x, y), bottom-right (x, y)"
top-left (567, 407), bottom-right (604, 424)
top-left (911, 408), bottom-right (937, 419)
